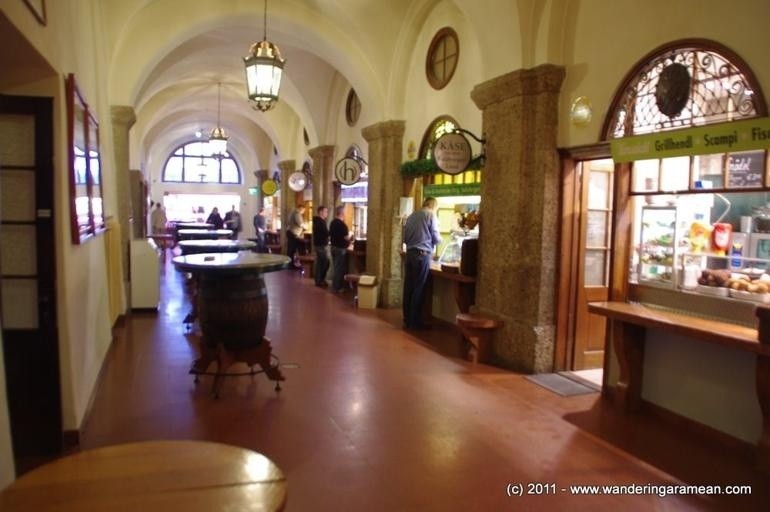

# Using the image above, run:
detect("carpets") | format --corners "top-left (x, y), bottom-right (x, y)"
top-left (522, 373), bottom-right (598, 397)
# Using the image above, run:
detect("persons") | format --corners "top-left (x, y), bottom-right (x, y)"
top-left (151, 203), bottom-right (167, 250)
top-left (329, 206), bottom-right (354, 293)
top-left (254, 208), bottom-right (267, 251)
top-left (401, 198), bottom-right (443, 329)
top-left (224, 205), bottom-right (242, 240)
top-left (287, 205), bottom-right (308, 270)
top-left (206, 207), bottom-right (223, 229)
top-left (312, 206), bottom-right (331, 288)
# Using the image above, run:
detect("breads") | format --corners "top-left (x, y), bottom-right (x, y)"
top-left (697, 269), bottom-right (770, 294)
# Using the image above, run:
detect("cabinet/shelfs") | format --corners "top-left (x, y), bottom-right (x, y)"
top-left (636, 206), bottom-right (689, 290)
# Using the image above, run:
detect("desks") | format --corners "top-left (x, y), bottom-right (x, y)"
top-left (144, 220), bottom-right (360, 401)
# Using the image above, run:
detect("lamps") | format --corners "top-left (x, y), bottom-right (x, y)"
top-left (242, 1), bottom-right (286, 111)
top-left (208, 82), bottom-right (230, 161)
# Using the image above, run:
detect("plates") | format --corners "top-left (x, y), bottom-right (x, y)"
top-left (643, 176), bottom-right (673, 205)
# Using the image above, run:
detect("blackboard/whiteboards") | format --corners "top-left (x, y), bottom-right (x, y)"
top-left (720, 149), bottom-right (766, 191)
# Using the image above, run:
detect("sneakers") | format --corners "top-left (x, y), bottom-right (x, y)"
top-left (336, 288), bottom-right (350, 293)
top-left (316, 281), bottom-right (328, 287)
top-left (404, 320), bottom-right (431, 330)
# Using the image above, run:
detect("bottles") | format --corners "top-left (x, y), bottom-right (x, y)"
top-left (711, 222), bottom-right (733, 256)
top-left (689, 212), bottom-right (709, 251)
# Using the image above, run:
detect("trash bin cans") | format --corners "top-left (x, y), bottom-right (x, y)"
top-left (357, 275), bottom-right (379, 309)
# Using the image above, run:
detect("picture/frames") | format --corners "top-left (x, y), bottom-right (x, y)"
top-left (67, 70), bottom-right (109, 245)
